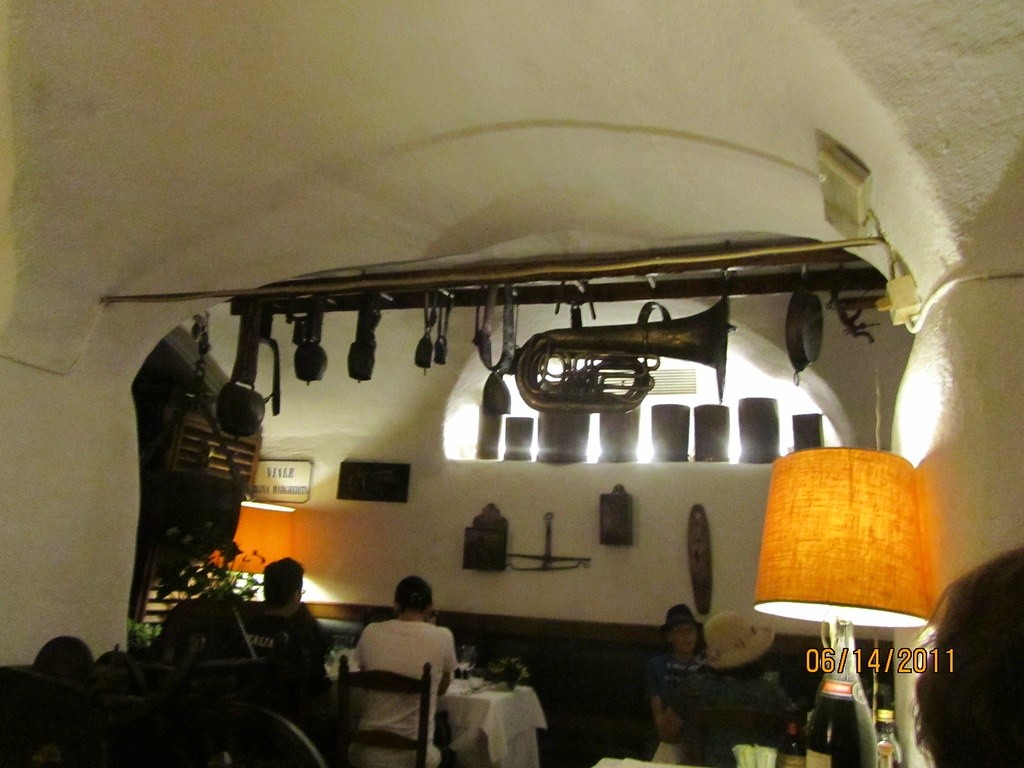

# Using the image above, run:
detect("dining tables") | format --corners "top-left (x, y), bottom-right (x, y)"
top-left (322, 667), bottom-right (550, 768)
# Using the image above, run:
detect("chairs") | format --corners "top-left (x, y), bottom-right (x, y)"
top-left (331, 653), bottom-right (433, 767)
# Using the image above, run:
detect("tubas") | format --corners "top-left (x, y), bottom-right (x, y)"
top-left (514, 294), bottom-right (729, 414)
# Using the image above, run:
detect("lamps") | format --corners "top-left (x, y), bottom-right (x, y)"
top-left (750, 444), bottom-right (935, 627)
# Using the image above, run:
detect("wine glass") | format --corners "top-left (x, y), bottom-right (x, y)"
top-left (455, 645), bottom-right (476, 690)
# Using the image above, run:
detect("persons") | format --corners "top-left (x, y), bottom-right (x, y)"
top-left (33, 628), bottom-right (205, 768)
top-left (201, 559), bottom-right (325, 670)
top-left (910, 547), bottom-right (1024, 768)
top-left (638, 604), bottom-right (705, 721)
top-left (657, 611), bottom-right (785, 768)
top-left (345, 576), bottom-right (457, 768)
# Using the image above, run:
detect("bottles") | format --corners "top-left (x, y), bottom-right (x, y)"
top-left (874, 710), bottom-right (902, 768)
top-left (805, 619), bottom-right (878, 768)
top-left (775, 723), bottom-right (805, 768)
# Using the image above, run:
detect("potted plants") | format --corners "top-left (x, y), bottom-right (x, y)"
top-left (125, 616), bottom-right (162, 659)
top-left (484, 655), bottom-right (530, 691)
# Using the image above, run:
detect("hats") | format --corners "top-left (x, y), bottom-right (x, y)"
top-left (659, 604), bottom-right (703, 635)
top-left (703, 610), bottom-right (774, 669)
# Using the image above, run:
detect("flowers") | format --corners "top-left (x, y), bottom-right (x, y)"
top-left (173, 545), bottom-right (264, 616)
